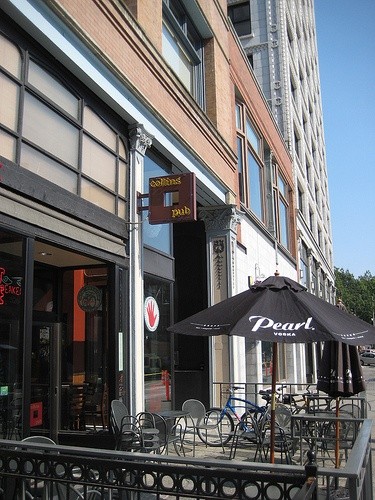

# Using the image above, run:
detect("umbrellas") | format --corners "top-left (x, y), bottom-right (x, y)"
top-left (167, 270), bottom-right (375, 470)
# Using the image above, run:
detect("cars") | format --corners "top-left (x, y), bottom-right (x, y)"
top-left (359, 349), bottom-right (375, 366)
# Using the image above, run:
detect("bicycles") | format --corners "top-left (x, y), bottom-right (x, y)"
top-left (196, 385), bottom-right (361, 451)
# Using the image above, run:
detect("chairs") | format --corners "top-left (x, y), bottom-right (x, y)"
top-left (13, 433), bottom-right (61, 475)
top-left (302, 403), bottom-right (363, 467)
top-left (182, 399), bottom-right (225, 454)
top-left (223, 406), bottom-right (269, 461)
top-left (262, 402), bottom-right (299, 459)
top-left (108, 398), bottom-right (181, 483)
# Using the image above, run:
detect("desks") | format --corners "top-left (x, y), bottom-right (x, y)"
top-left (290, 412), bottom-right (365, 464)
top-left (151, 411), bottom-right (196, 458)
